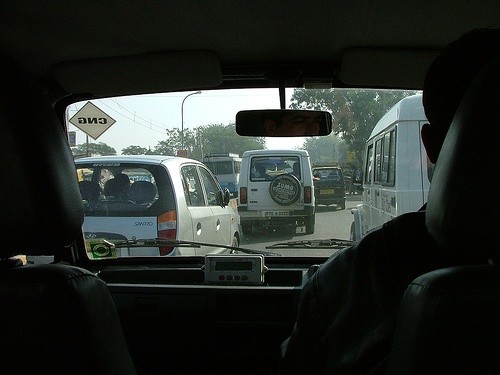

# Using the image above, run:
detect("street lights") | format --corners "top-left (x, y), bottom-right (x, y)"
top-left (181, 91), bottom-right (202, 156)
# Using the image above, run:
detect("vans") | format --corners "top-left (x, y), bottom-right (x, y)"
top-left (350, 94), bottom-right (430, 242)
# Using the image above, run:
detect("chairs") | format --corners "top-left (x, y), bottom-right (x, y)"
top-left (106, 178), bottom-right (124, 197)
top-left (128, 181), bottom-right (156, 207)
top-left (382, 94), bottom-right (500, 375)
top-left (290, 162), bottom-right (300, 177)
top-left (76, 182), bottom-right (100, 205)
top-left (0, 95), bottom-right (138, 375)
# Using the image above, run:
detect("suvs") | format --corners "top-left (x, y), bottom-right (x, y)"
top-left (237, 149), bottom-right (316, 235)
top-left (312, 162), bottom-right (347, 210)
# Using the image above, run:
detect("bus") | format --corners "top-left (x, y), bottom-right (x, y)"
top-left (203, 153), bottom-right (242, 198)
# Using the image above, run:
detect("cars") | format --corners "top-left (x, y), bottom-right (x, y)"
top-left (73, 155), bottom-right (241, 258)
